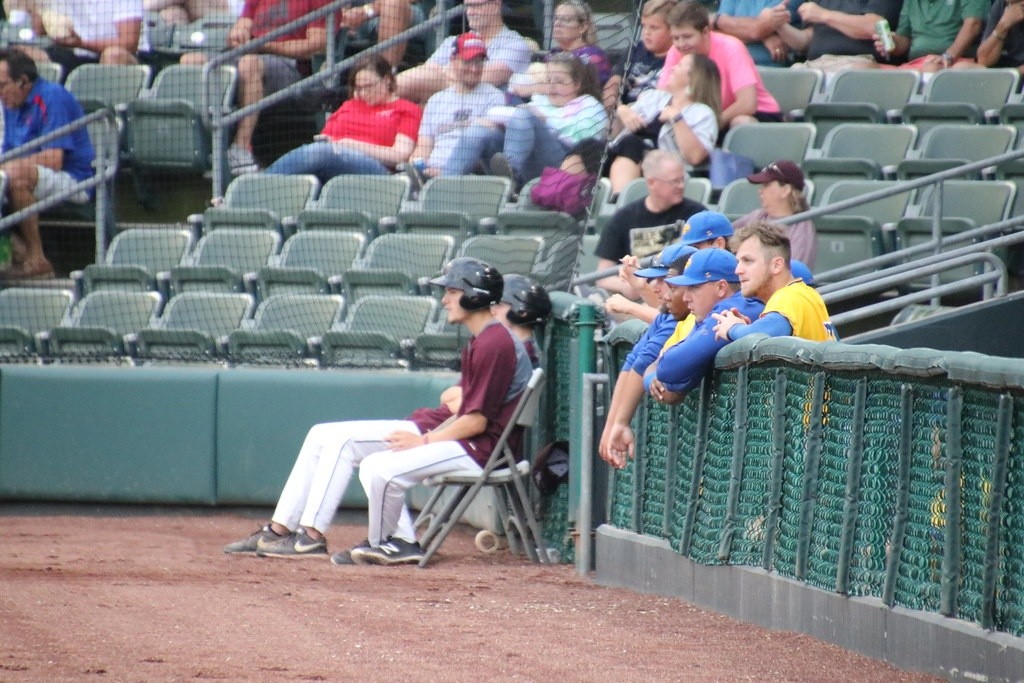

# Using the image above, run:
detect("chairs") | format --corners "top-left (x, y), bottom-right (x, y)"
top-left (413, 368), bottom-right (550, 570)
top-left (0, 16), bottom-right (1024, 363)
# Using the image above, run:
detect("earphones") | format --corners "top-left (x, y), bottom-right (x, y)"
top-left (19, 79), bottom-right (26, 87)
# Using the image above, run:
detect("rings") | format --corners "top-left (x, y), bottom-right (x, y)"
top-left (775, 48), bottom-right (781, 54)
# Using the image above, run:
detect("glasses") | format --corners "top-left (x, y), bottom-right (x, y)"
top-left (654, 175), bottom-right (689, 186)
top-left (354, 80), bottom-right (380, 93)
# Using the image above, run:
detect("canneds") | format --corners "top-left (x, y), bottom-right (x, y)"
top-left (412, 157), bottom-right (432, 183)
top-left (874, 19), bottom-right (895, 53)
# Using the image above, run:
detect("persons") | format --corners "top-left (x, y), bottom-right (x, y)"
top-left (0, 0), bottom-right (1024, 288)
top-left (222, 273), bottom-right (552, 560)
top-left (330, 256), bottom-right (533, 565)
top-left (589, 149), bottom-right (843, 468)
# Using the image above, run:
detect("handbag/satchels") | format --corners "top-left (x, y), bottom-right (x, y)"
top-left (530, 166), bottom-right (597, 221)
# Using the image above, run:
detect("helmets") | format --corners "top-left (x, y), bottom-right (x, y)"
top-left (501, 274), bottom-right (553, 325)
top-left (429, 257), bottom-right (504, 312)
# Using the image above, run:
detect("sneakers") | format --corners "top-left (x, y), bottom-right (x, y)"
top-left (223, 523), bottom-right (290, 558)
top-left (331, 537), bottom-right (426, 566)
top-left (406, 164), bottom-right (434, 193)
top-left (0, 255), bottom-right (54, 282)
top-left (202, 143), bottom-right (259, 178)
top-left (256, 527), bottom-right (328, 561)
top-left (490, 153), bottom-right (523, 202)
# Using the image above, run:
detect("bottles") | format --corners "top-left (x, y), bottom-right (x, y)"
top-left (533, 549), bottom-right (561, 564)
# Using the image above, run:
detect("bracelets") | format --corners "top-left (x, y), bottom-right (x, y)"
top-left (942, 52), bottom-right (955, 63)
top-left (422, 434), bottom-right (429, 445)
top-left (669, 112), bottom-right (685, 124)
top-left (991, 29), bottom-right (1008, 42)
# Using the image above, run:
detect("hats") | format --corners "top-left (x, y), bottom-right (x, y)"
top-left (747, 160), bottom-right (805, 191)
top-left (663, 247), bottom-right (740, 286)
top-left (633, 244), bottom-right (699, 278)
top-left (675, 211), bottom-right (734, 245)
top-left (451, 32), bottom-right (487, 60)
